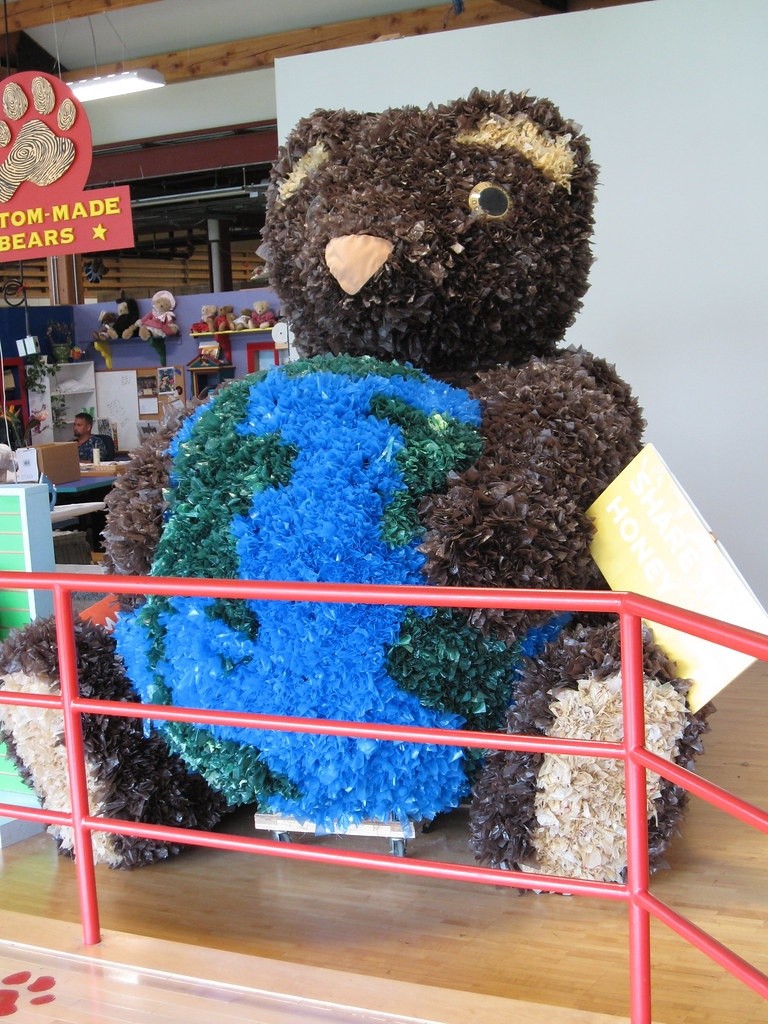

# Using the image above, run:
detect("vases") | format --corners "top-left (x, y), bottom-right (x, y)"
top-left (51, 344), bottom-right (70, 363)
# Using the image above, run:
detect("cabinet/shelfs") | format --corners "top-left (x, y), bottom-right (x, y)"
top-left (27, 361), bottom-right (98, 443)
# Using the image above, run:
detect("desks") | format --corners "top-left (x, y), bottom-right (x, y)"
top-left (52, 474), bottom-right (117, 492)
top-left (51, 500), bottom-right (106, 524)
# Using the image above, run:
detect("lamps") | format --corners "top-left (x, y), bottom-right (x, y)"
top-left (64, 12), bottom-right (167, 103)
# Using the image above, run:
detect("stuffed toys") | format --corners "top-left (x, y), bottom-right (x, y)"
top-left (92, 289), bottom-right (290, 344)
top-left (4, 90), bottom-right (718, 900)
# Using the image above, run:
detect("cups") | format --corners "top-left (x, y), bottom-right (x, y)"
top-left (93, 448), bottom-right (100, 464)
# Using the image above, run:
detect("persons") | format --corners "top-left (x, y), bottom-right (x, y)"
top-left (71, 412), bottom-right (107, 465)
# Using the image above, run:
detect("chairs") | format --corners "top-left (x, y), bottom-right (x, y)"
top-left (94, 434), bottom-right (116, 461)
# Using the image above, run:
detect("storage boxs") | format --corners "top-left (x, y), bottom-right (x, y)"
top-left (18, 440), bottom-right (80, 484)
